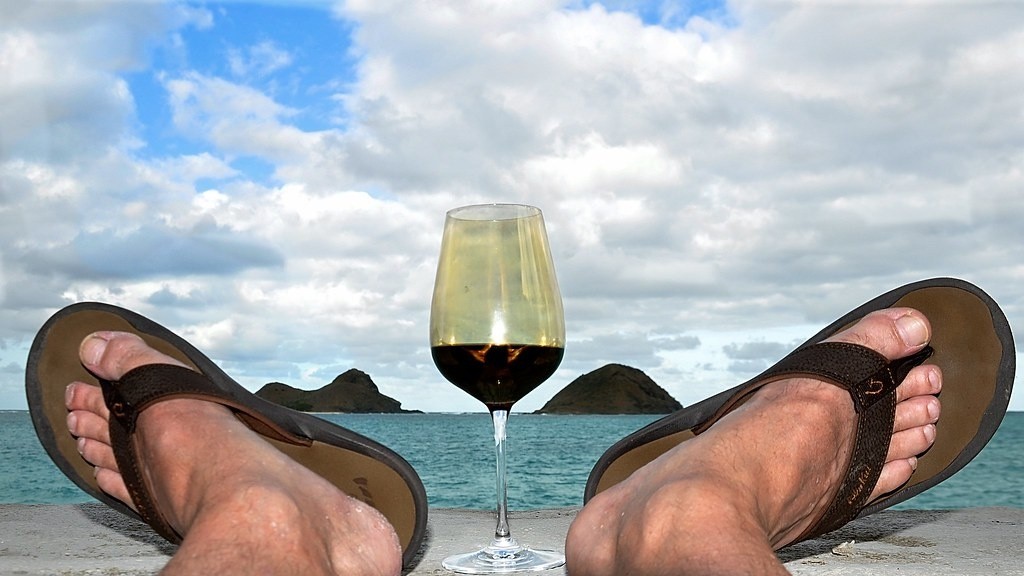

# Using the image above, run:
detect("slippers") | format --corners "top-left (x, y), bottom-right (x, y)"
top-left (25, 302), bottom-right (433, 576)
top-left (584, 278), bottom-right (1016, 564)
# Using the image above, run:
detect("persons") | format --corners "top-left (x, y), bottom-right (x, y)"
top-left (25, 278), bottom-right (1016, 576)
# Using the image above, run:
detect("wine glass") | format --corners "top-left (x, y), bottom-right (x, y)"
top-left (430, 204), bottom-right (566, 575)
